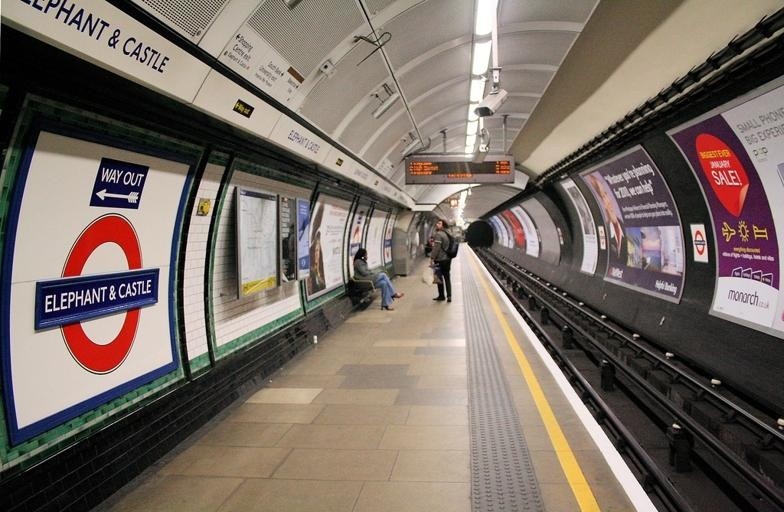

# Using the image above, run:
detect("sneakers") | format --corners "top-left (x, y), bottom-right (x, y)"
top-left (447, 298), bottom-right (451, 301)
top-left (433, 296), bottom-right (445, 300)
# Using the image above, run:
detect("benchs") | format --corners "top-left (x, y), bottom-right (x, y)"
top-left (350, 271), bottom-right (388, 310)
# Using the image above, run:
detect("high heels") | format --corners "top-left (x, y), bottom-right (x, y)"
top-left (391, 292), bottom-right (404, 301)
top-left (381, 305), bottom-right (393, 310)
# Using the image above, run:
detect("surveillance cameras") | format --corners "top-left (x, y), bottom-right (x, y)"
top-left (472, 144), bottom-right (491, 164)
top-left (474, 88), bottom-right (509, 117)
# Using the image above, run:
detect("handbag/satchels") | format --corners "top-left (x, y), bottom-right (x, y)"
top-left (421, 264), bottom-right (443, 285)
top-left (448, 239), bottom-right (458, 258)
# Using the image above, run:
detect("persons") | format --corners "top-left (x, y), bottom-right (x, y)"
top-left (590, 177), bottom-right (625, 252)
top-left (430, 219), bottom-right (458, 302)
top-left (425, 236), bottom-right (434, 249)
top-left (309, 242), bottom-right (325, 293)
top-left (354, 246), bottom-right (404, 311)
